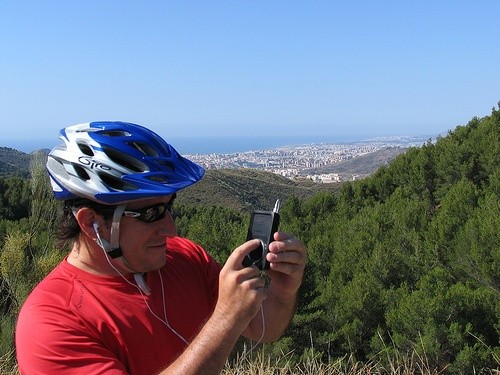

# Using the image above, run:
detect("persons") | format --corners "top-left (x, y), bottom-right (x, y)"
top-left (16, 121), bottom-right (307, 374)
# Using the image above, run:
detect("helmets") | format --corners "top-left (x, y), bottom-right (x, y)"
top-left (45, 120), bottom-right (206, 206)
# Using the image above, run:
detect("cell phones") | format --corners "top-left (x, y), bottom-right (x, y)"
top-left (244, 210), bottom-right (280, 271)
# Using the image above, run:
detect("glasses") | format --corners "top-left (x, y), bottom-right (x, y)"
top-left (78, 192), bottom-right (176, 223)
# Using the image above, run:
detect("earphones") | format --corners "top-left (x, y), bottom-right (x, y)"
top-left (93, 221), bottom-right (100, 240)
